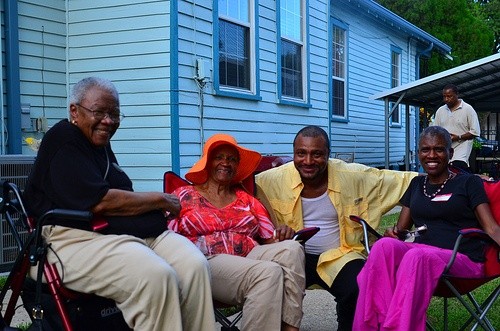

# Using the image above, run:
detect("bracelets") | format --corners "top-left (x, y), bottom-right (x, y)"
top-left (458, 134), bottom-right (462, 142)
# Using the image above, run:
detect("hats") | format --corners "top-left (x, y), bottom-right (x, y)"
top-left (184, 134), bottom-right (261, 186)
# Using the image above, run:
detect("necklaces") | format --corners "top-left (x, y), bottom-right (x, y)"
top-left (424, 170), bottom-right (453, 197)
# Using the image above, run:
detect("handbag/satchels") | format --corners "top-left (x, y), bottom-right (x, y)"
top-left (0, 230), bottom-right (54, 331)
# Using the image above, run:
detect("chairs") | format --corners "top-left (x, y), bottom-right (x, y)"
top-left (349, 180), bottom-right (500, 331)
top-left (0, 178), bottom-right (172, 331)
top-left (163, 171), bottom-right (320, 331)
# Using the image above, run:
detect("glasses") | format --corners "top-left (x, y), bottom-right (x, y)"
top-left (75, 103), bottom-right (125, 123)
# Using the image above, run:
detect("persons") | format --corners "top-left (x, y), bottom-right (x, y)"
top-left (435, 84), bottom-right (480, 173)
top-left (166, 133), bottom-right (306, 331)
top-left (25, 78), bottom-right (216, 331)
top-left (255, 125), bottom-right (428, 331)
top-left (353, 126), bottom-right (500, 331)
